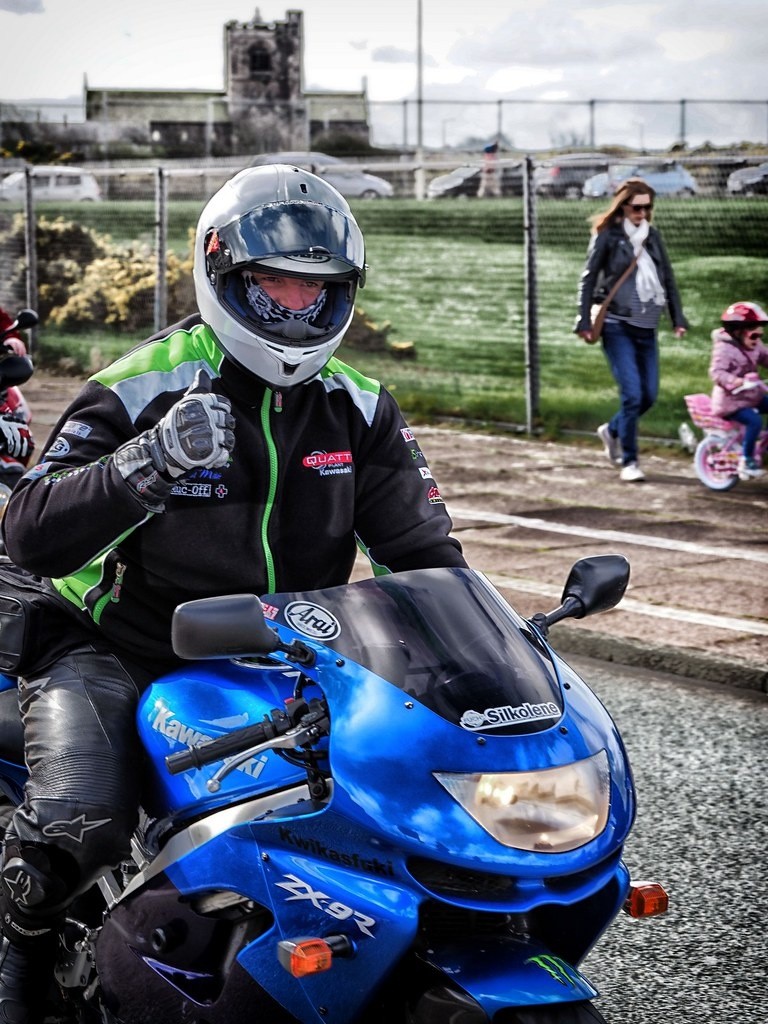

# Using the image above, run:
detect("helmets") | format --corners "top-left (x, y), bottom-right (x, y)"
top-left (721, 301), bottom-right (768, 327)
top-left (192, 165), bottom-right (368, 382)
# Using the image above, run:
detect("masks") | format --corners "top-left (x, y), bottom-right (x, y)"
top-left (243, 270), bottom-right (328, 324)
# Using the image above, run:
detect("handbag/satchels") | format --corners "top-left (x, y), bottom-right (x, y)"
top-left (574, 303), bottom-right (606, 343)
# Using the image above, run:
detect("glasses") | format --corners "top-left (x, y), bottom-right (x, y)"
top-left (627, 203), bottom-right (652, 211)
top-left (743, 331), bottom-right (764, 340)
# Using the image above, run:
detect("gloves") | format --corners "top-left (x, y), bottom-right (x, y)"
top-left (109, 370), bottom-right (236, 510)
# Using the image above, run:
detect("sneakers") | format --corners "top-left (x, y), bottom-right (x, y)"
top-left (619, 465), bottom-right (645, 480)
top-left (738, 459), bottom-right (767, 477)
top-left (597, 423), bottom-right (617, 458)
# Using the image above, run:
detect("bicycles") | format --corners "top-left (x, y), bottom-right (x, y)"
top-left (682, 372), bottom-right (768, 494)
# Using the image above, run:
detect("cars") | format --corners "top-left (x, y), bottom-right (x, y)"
top-left (243, 150), bottom-right (396, 200)
top-left (426, 158), bottom-right (536, 201)
top-left (529, 151), bottom-right (618, 201)
top-left (0, 164), bottom-right (103, 204)
top-left (725, 160), bottom-right (768, 196)
top-left (582, 155), bottom-right (700, 200)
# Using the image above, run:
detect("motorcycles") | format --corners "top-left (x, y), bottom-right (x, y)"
top-left (0, 552), bottom-right (670, 1024)
top-left (0, 308), bottom-right (40, 518)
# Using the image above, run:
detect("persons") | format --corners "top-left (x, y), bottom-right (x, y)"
top-left (0, 162), bottom-right (469, 1024)
top-left (708, 300), bottom-right (768, 481)
top-left (0, 306), bottom-right (36, 490)
top-left (571, 179), bottom-right (691, 479)
